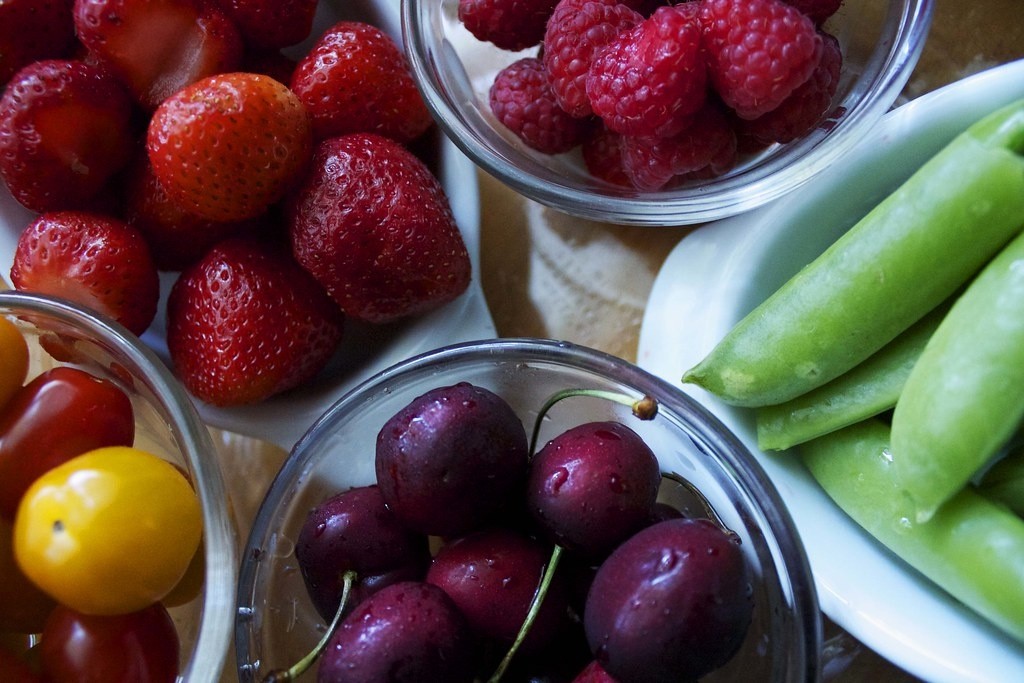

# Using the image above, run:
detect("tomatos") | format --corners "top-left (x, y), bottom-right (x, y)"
top-left (0, 310), bottom-right (202, 683)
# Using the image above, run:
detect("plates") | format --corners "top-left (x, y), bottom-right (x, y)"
top-left (638, 60), bottom-right (1024, 683)
top-left (0, 0), bottom-right (482, 436)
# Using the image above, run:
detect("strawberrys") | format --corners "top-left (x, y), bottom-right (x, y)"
top-left (1, 0), bottom-right (473, 407)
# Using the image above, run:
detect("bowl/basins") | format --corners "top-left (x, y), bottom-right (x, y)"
top-left (401, 0), bottom-right (929, 225)
top-left (233, 341), bottom-right (816, 683)
top-left (0, 292), bottom-right (234, 683)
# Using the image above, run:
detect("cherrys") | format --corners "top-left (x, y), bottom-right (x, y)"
top-left (262, 381), bottom-right (753, 683)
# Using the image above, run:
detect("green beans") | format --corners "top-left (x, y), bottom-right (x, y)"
top-left (678, 94), bottom-right (1023, 647)
